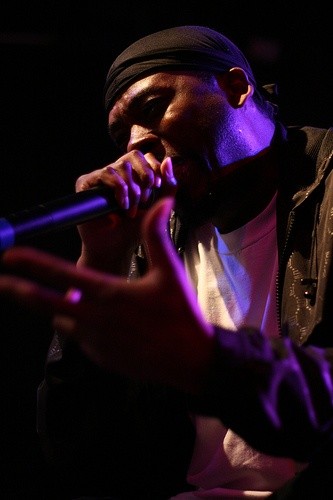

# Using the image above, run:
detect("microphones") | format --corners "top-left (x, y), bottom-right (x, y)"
top-left (0, 184), bottom-right (161, 250)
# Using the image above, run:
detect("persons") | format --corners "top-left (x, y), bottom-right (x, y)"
top-left (0, 24), bottom-right (333, 500)
